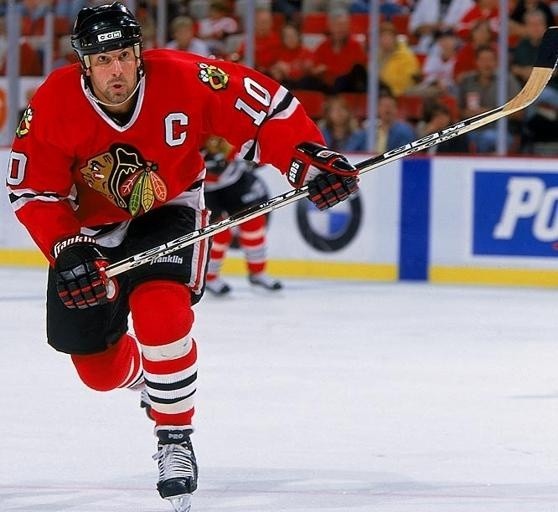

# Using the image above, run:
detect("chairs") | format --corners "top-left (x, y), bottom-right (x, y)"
top-left (0, 0), bottom-right (558, 157)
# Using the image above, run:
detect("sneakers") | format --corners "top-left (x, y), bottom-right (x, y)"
top-left (153, 424), bottom-right (198, 498)
top-left (205, 278), bottom-right (231, 295)
top-left (251, 273), bottom-right (283, 290)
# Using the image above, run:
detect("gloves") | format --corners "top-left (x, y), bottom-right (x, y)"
top-left (289, 137), bottom-right (360, 211)
top-left (51, 235), bottom-right (119, 311)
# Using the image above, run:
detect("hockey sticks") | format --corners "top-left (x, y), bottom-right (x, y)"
top-left (105, 27), bottom-right (558, 277)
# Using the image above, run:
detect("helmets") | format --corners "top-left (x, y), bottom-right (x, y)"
top-left (69, 1), bottom-right (141, 78)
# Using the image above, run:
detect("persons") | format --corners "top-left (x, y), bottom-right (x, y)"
top-left (0, 0), bottom-right (558, 162)
top-left (6, 2), bottom-right (362, 501)
top-left (196, 136), bottom-right (284, 295)
